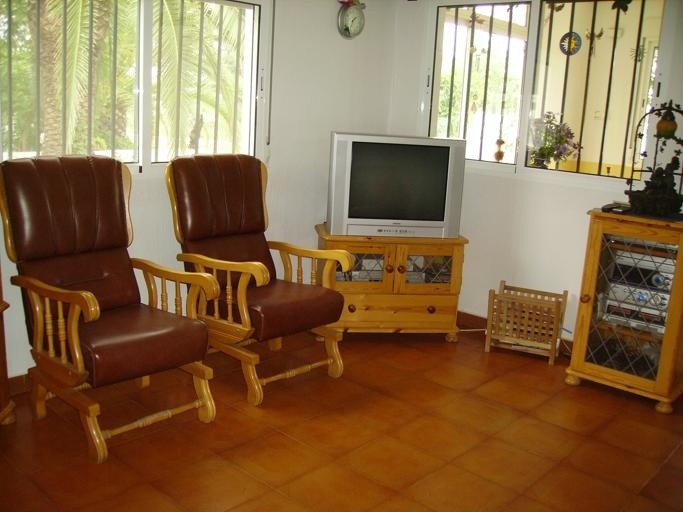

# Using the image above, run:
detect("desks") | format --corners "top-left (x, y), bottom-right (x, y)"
top-left (0, 283), bottom-right (16, 426)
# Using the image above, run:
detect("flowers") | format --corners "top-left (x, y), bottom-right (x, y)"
top-left (526, 111), bottom-right (583, 161)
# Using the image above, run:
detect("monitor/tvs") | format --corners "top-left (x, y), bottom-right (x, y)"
top-left (326, 132), bottom-right (466, 238)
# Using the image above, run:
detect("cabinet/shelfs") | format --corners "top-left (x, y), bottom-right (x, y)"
top-left (564, 207), bottom-right (683, 413)
top-left (484, 280), bottom-right (568, 367)
top-left (315, 225), bottom-right (469, 342)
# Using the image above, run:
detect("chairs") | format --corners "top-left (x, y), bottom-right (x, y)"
top-left (165, 154), bottom-right (358, 406)
top-left (0, 154), bottom-right (221, 464)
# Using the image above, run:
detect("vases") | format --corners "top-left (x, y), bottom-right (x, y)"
top-left (530, 159), bottom-right (548, 168)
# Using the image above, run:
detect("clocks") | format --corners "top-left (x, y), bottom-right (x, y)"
top-left (338, 1), bottom-right (365, 39)
